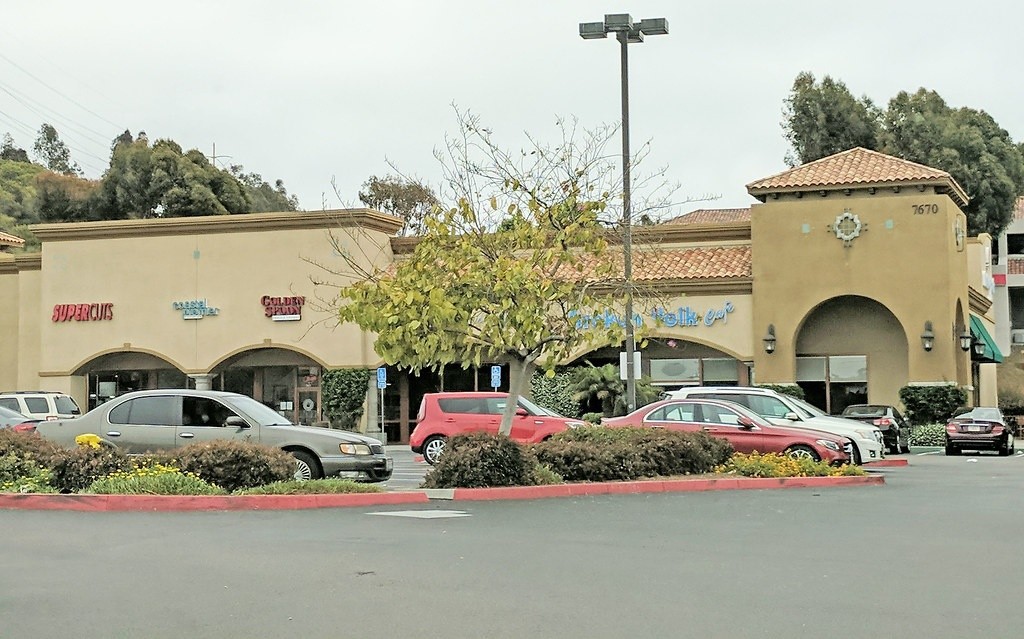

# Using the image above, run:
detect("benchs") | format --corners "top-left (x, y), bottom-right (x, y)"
top-left (850, 410), bottom-right (884, 416)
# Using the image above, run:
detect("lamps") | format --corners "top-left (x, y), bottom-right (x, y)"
top-left (952, 322), bottom-right (973, 353)
top-left (970, 332), bottom-right (986, 357)
top-left (920, 321), bottom-right (935, 353)
top-left (762, 324), bottom-right (776, 354)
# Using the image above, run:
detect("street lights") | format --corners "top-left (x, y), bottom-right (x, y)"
top-left (578, 12), bottom-right (668, 413)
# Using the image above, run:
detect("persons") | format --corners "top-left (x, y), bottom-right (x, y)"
top-left (206, 407), bottom-right (232, 427)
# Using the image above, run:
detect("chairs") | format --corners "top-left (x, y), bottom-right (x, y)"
top-left (709, 413), bottom-right (721, 422)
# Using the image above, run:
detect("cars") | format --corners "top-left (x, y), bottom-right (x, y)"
top-left (33, 389), bottom-right (394, 484)
top-left (599, 400), bottom-right (853, 471)
top-left (0, 404), bottom-right (46, 437)
top-left (945, 406), bottom-right (1017, 456)
top-left (409, 391), bottom-right (592, 467)
top-left (840, 404), bottom-right (912, 454)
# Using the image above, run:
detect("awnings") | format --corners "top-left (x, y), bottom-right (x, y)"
top-left (969, 313), bottom-right (1003, 364)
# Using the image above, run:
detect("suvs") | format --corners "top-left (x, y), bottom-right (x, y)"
top-left (0, 391), bottom-right (82, 429)
top-left (662, 386), bottom-right (887, 467)
